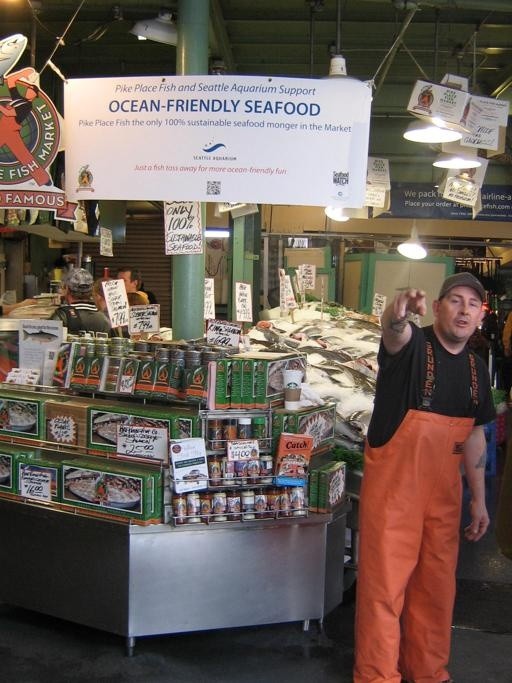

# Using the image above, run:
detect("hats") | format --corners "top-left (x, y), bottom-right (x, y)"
top-left (62, 268), bottom-right (92, 292)
top-left (437, 272), bottom-right (486, 302)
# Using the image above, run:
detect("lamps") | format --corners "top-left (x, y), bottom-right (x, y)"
top-left (320, 0), bottom-right (359, 83)
top-left (400, 7), bottom-right (464, 144)
top-left (430, 139), bottom-right (483, 171)
top-left (128, 8), bottom-right (185, 49)
top-left (323, 203), bottom-right (349, 223)
top-left (396, 219), bottom-right (427, 261)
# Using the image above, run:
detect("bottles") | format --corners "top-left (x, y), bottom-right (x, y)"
top-left (221, 454), bottom-right (234, 487)
top-left (279, 485), bottom-right (293, 518)
top-left (199, 492), bottom-right (212, 524)
top-left (291, 485), bottom-right (305, 516)
top-left (227, 488), bottom-right (242, 521)
top-left (212, 490), bottom-right (227, 522)
top-left (67, 334), bottom-right (239, 367)
top-left (260, 452), bottom-right (273, 484)
top-left (267, 486), bottom-right (280, 519)
top-left (238, 417), bottom-right (254, 441)
top-left (252, 417), bottom-right (268, 450)
top-left (241, 488), bottom-right (257, 523)
top-left (185, 493), bottom-right (201, 526)
top-left (209, 419), bottom-right (222, 450)
top-left (222, 418), bottom-right (237, 450)
top-left (172, 493), bottom-right (188, 525)
top-left (255, 488), bottom-right (268, 519)
top-left (234, 459), bottom-right (248, 486)
top-left (207, 454), bottom-right (221, 487)
top-left (248, 459), bottom-right (260, 484)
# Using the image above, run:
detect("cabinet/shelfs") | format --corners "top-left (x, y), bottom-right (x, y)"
top-left (0, 381), bottom-right (350, 657)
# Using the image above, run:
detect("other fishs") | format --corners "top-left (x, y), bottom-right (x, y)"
top-left (249, 321), bottom-right (385, 452)
top-left (23, 330), bottom-right (58, 345)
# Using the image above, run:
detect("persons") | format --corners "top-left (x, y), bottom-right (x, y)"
top-left (48, 267), bottom-right (111, 339)
top-left (354, 270), bottom-right (495, 683)
top-left (0, 298), bottom-right (37, 318)
top-left (116, 265), bottom-right (157, 338)
